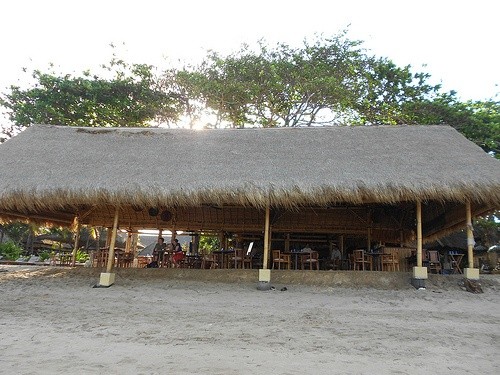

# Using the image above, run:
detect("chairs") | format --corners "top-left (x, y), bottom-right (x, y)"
top-left (138, 247), bottom-right (255, 269)
top-left (272, 249), bottom-right (320, 271)
top-left (428, 249), bottom-right (443, 274)
top-left (347, 249), bottom-right (400, 272)
top-left (60, 248), bottom-right (135, 268)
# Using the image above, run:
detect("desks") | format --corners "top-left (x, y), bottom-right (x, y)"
top-left (100, 247), bottom-right (122, 265)
top-left (367, 251), bottom-right (393, 271)
top-left (448, 253), bottom-right (465, 276)
top-left (155, 250), bottom-right (170, 267)
top-left (214, 250), bottom-right (236, 269)
top-left (285, 251), bottom-right (310, 269)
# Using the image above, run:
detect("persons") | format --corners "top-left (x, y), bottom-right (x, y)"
top-left (330, 243), bottom-right (342, 264)
top-left (171, 239), bottom-right (184, 269)
top-left (153, 237), bottom-right (166, 261)
top-left (303, 243), bottom-right (312, 252)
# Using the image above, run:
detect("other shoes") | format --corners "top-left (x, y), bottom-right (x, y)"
top-left (176, 265), bottom-right (179, 268)
